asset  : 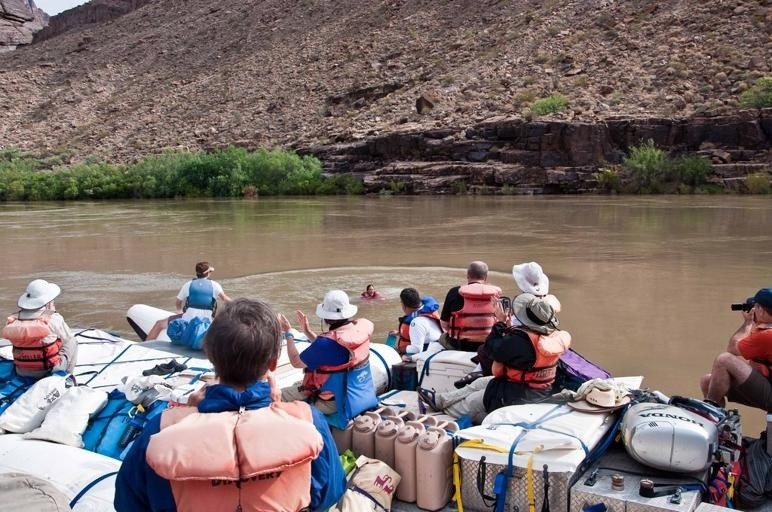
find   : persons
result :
[439,259,504,352]
[144,261,234,341]
[112,297,349,511]
[453,260,550,389]
[359,283,384,301]
[273,288,374,417]
[416,291,559,424]
[387,287,446,363]
[698,287,771,416]
[6,278,80,378]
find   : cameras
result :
[731,297,755,313]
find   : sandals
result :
[417,386,437,411]
[454,371,483,388]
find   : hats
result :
[567,387,631,414]
[16,278,62,310]
[315,289,358,321]
[745,287,772,307]
[511,260,560,335]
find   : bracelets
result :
[284,330,295,339]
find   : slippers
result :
[142,359,188,376]
